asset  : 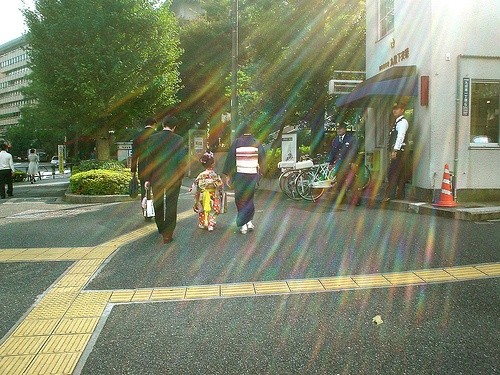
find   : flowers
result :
[204,150,214,159]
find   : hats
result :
[392,102,406,108]
[335,122,347,129]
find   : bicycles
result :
[277,151,372,202]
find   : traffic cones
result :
[431,163,461,208]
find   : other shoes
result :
[240,229,247,234]
[163,233,172,243]
[208,226,213,231]
[199,223,205,228]
[248,224,255,229]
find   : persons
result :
[381,100,409,203]
[130,117,159,221]
[27,148,39,183]
[0,144,16,199]
[327,119,361,207]
[223,122,266,235]
[144,115,185,243]
[190,149,231,232]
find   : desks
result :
[14,165,54,183]
[50,163,74,179]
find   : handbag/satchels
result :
[142,188,155,218]
[129,173,138,198]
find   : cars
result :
[51,156,66,166]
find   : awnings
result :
[334,64,419,109]
